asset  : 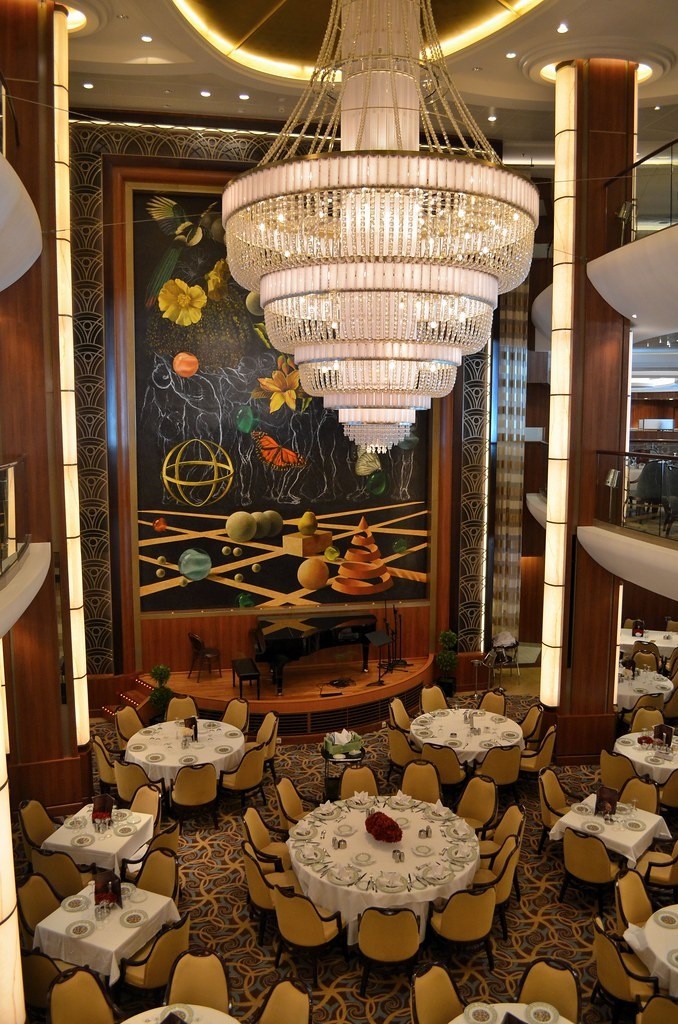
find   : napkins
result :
[380,871,402,886]
[455,818,469,837]
[316,799,337,816]
[425,860,444,880]
[295,819,312,836]
[455,844,471,858]
[334,866,353,881]
[351,790,369,804]
[393,789,411,804]
[302,846,319,861]
[432,798,448,817]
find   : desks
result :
[492,637,520,677]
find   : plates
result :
[615,805,632,815]
[625,819,646,831]
[179,755,197,765]
[526,1002,559,1024]
[112,810,129,821]
[644,756,665,765]
[667,949,678,969]
[215,745,233,754]
[225,730,241,738]
[159,1003,193,1024]
[204,722,218,729]
[463,1002,497,1024]
[64,816,87,829]
[571,802,593,815]
[129,743,146,752]
[289,796,478,892]
[63,895,89,912]
[653,911,678,929]
[145,753,164,762]
[581,821,604,834]
[121,882,137,898]
[66,919,95,938]
[72,833,95,847]
[616,738,635,746]
[139,728,156,736]
[634,688,649,695]
[656,684,671,691]
[416,709,520,749]
[114,824,137,836]
[120,910,148,927]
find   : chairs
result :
[662,465,678,537]
[14,617,678,1024]
[628,460,664,522]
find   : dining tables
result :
[615,667,675,711]
[287,794,482,962]
[623,904,678,998]
[611,731,678,784]
[409,707,526,769]
[548,792,672,872]
[125,717,246,807]
[31,879,181,1016]
[619,628,678,663]
[448,1003,573,1024]
[121,1003,242,1024]
[41,802,154,882]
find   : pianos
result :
[249,614,378,696]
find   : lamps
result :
[220,0,540,458]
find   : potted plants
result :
[436,629,458,699]
[148,663,172,726]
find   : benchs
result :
[231,658,260,699]
